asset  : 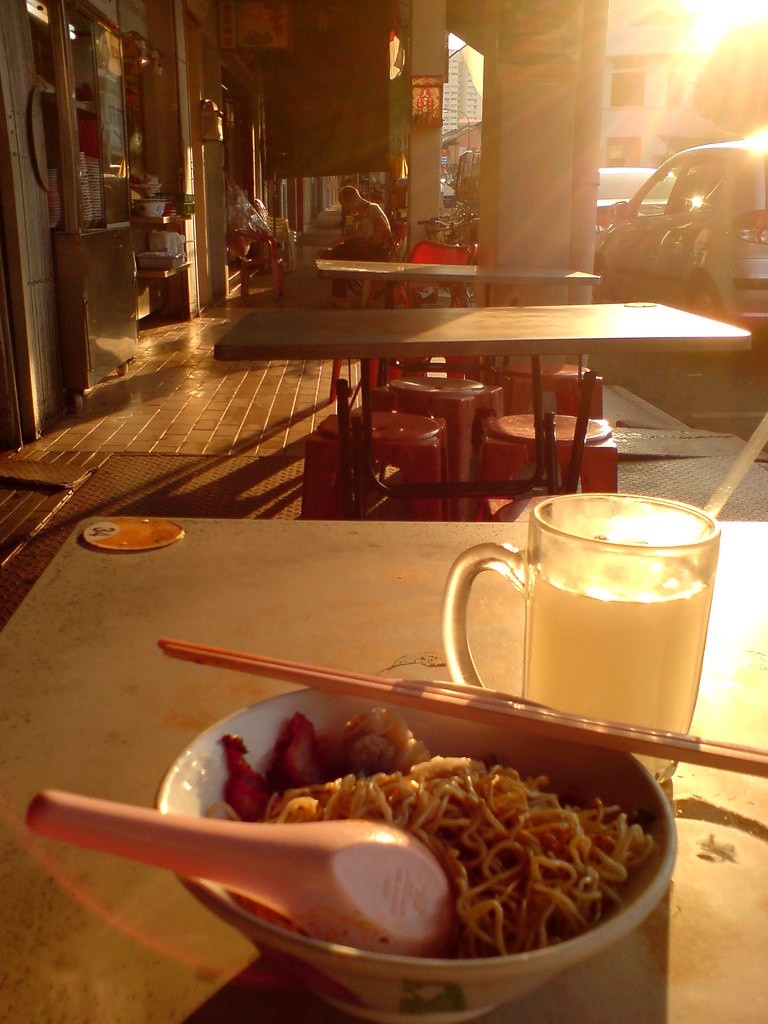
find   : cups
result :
[441,492,721,785]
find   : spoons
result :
[24,786,457,959]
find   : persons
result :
[321,186,394,309]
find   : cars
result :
[595,137,767,330]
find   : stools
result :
[295,356,619,523]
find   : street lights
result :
[441,108,471,150]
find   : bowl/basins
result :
[153,680,677,1024]
[47,151,99,228]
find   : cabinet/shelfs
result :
[132,215,193,279]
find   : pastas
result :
[202,762,653,957]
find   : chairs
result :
[341,223,409,308]
[408,239,477,310]
[227,226,284,298]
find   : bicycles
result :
[415,200,479,308]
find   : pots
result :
[133,198,168,216]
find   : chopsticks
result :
[158,637,768,780]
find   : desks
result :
[0,515,768,1024]
[218,303,754,523]
[313,258,600,306]
